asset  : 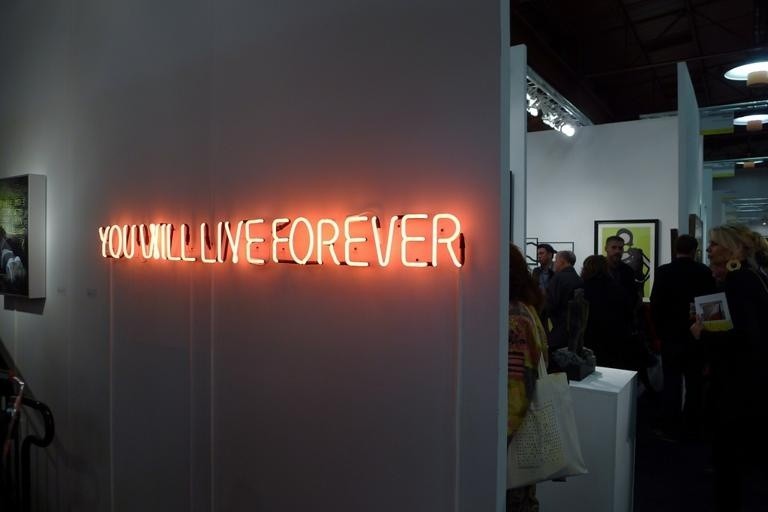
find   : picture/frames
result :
[591,216,661,308]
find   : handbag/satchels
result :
[505,297,590,492]
